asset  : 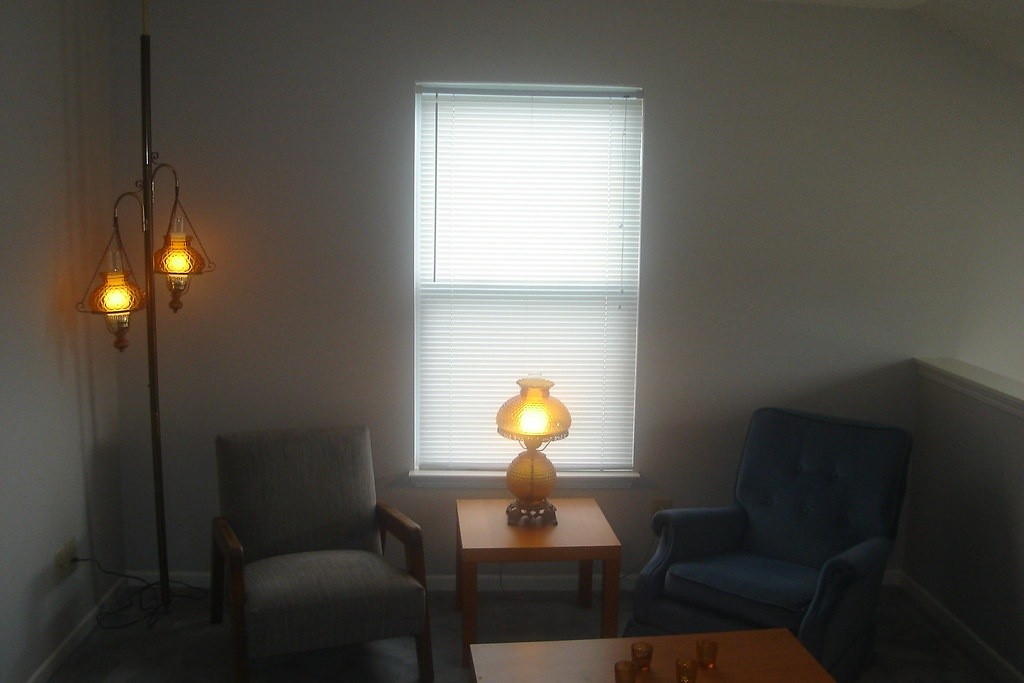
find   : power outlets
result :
[53,538,81,586]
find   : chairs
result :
[211,426,435,683]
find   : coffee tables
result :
[470,627,834,683]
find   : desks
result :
[456,498,622,667]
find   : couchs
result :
[622,407,911,673]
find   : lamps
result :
[77,34,216,606]
[497,378,571,526]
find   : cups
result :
[615,660,637,683]
[696,640,717,668]
[631,642,653,670]
[676,658,698,683]
